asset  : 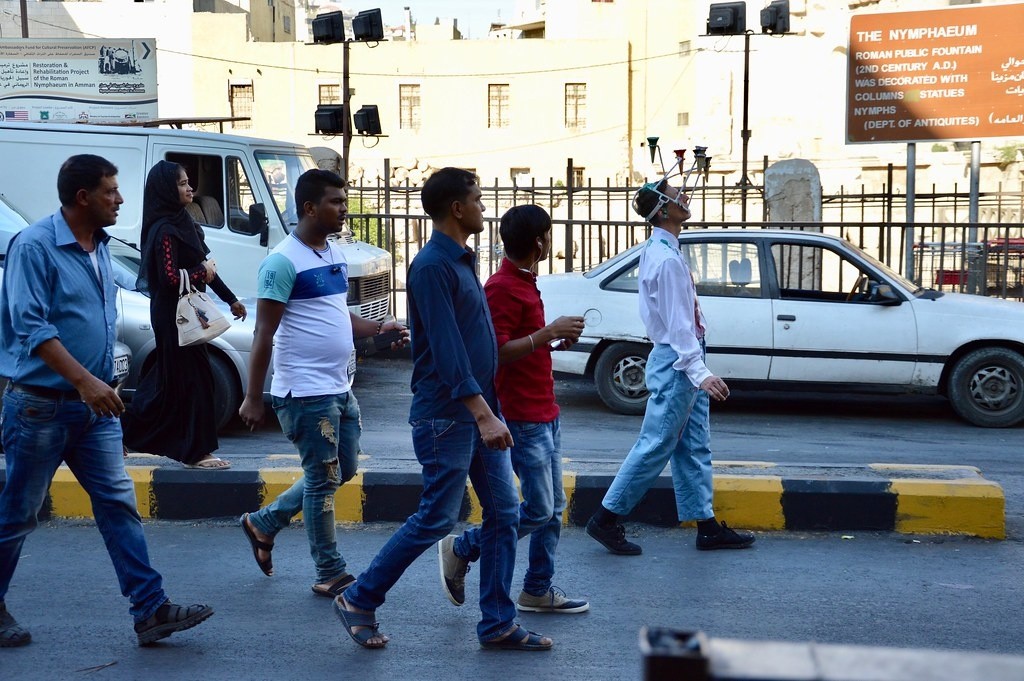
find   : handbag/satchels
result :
[176,269,232,347]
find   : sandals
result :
[134,599,214,645]
[0,616,31,647]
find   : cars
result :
[0,193,290,433]
[537,228,1023,428]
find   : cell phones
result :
[373,329,404,351]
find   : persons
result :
[121,160,247,469]
[436,204,592,615]
[237,170,409,597]
[0,153,216,649]
[333,169,553,651]
[586,180,757,558]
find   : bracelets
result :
[376,321,385,335]
[528,335,535,353]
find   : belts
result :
[14,378,119,401]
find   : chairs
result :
[193,196,223,226]
[729,258,753,297]
[186,202,208,225]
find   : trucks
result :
[0,119,393,355]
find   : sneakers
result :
[584,516,642,555]
[696,520,756,551]
[437,534,470,606]
[517,585,589,613]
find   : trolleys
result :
[909,240,985,296]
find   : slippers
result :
[311,574,357,597]
[479,624,553,651]
[240,512,274,576]
[183,458,231,469]
[331,595,386,648]
